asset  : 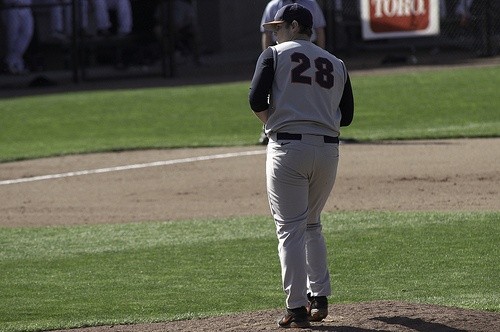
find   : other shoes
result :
[279,306,308,328]
[256,136,269,145]
[308,292,328,322]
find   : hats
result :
[263,3,314,35]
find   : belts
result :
[276,131,339,144]
